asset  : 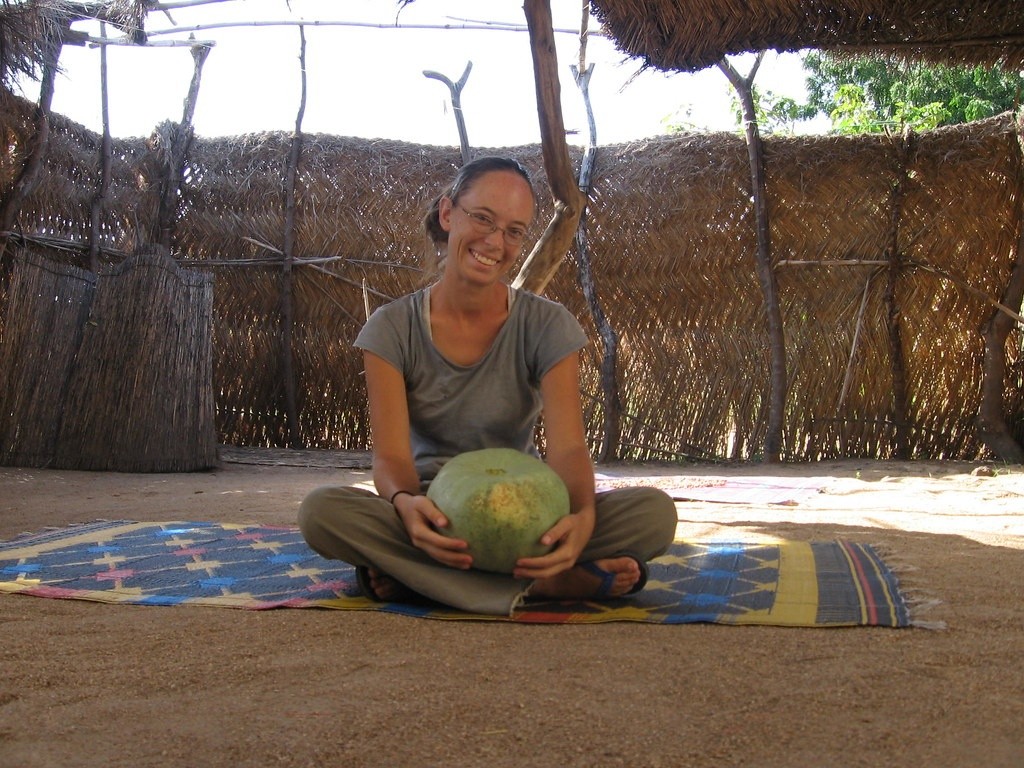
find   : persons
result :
[296,157,677,619]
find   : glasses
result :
[452,199,530,247]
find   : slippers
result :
[355,565,416,603]
[576,552,649,602]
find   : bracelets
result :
[391,491,415,504]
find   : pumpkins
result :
[425,446,570,573]
